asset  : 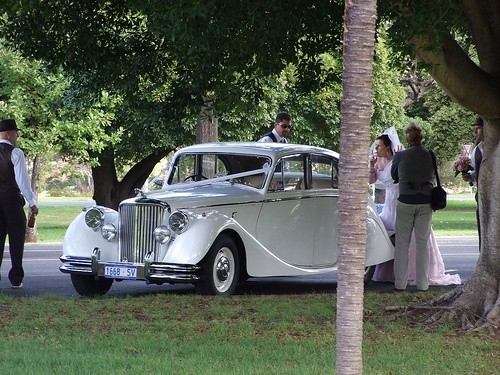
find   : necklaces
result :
[383,157,388,164]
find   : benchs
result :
[239,171,338,195]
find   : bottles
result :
[28,210,35,227]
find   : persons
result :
[257,113,292,184]
[0,119,38,288]
[367,117,483,290]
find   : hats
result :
[0,119,21,131]
[471,117,484,126]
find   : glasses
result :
[277,122,292,129]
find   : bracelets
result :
[370,170,376,174]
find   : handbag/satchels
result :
[431,186,446,211]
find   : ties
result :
[280,141,282,143]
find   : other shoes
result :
[11,278,24,289]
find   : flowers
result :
[453,156,475,186]
